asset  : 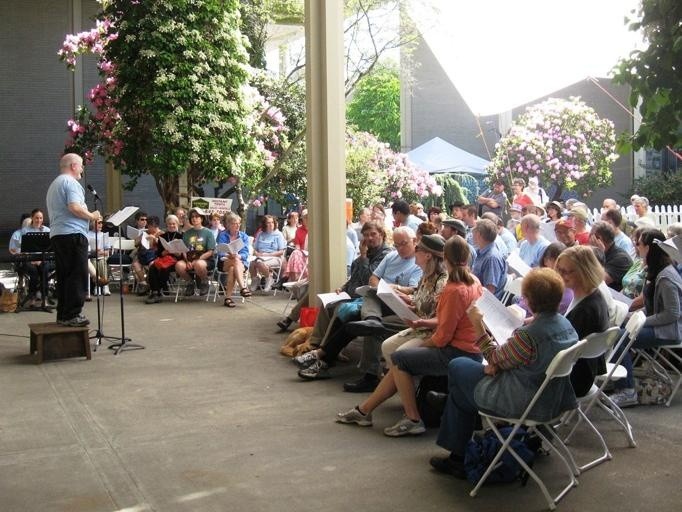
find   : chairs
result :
[341,270,682,474]
[469,338,589,510]
[5,215,310,319]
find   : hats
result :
[442,218,466,235]
[546,201,563,213]
[187,206,207,220]
[421,234,447,258]
[508,203,522,213]
[554,220,576,231]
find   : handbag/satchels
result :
[298,305,321,329]
[626,362,673,405]
[467,425,533,486]
[412,374,447,428]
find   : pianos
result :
[1,251,99,263]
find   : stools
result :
[24,321,92,366]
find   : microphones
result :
[87,184,101,200]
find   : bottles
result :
[122,281,129,293]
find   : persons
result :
[6,206,271,310]
[505,178,535,221]
[476,179,510,224]
[522,175,550,211]
[44,151,104,329]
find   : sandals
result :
[223,297,236,307]
[239,288,252,298]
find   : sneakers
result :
[184,281,210,298]
[293,349,331,380]
[383,415,427,437]
[56,313,91,327]
[344,377,383,393]
[250,276,274,293]
[429,450,465,480]
[278,316,293,329]
[135,283,165,304]
[335,404,375,428]
[94,278,112,297]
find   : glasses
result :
[140,218,148,221]
[603,387,638,408]
[394,240,408,247]
[414,245,425,253]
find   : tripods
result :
[25,250,58,312]
[108,227,147,354]
[89,201,131,351]
